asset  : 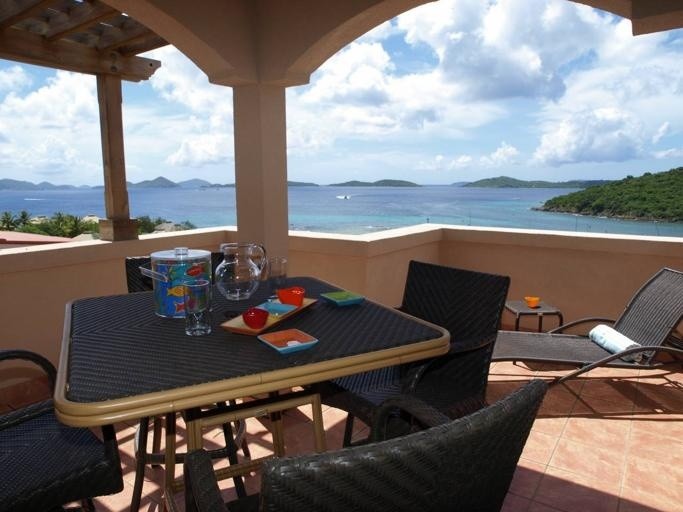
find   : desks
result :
[50,274,453,511]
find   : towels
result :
[588,323,642,362]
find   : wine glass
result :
[182,280,211,337]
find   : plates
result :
[219,292,365,355]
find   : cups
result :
[276,286,306,307]
[523,297,540,308]
[268,255,287,287]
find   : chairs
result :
[1,346,126,511]
[124,253,239,511]
[325,251,513,449]
[182,377,547,512]
[491,267,683,390]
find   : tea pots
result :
[213,241,266,302]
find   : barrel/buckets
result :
[139,248,214,319]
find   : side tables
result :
[505,298,563,365]
